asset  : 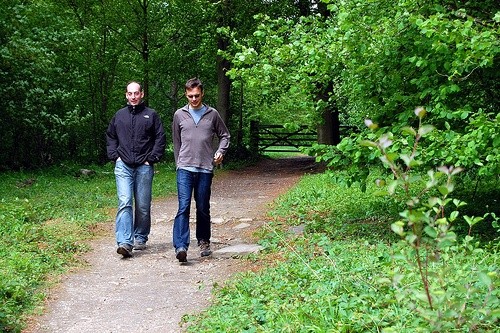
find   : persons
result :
[106,81,167,259]
[172,75,232,262]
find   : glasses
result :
[186,92,203,98]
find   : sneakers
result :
[199,240,211,256]
[117,245,132,257]
[176,248,187,262]
[133,238,146,250]
